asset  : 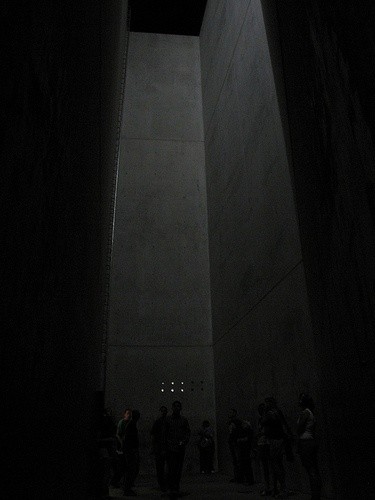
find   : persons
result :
[164,400,191,500]
[118,410,142,497]
[236,419,254,486]
[195,420,216,475]
[106,415,121,451]
[149,406,172,496]
[255,403,272,495]
[110,408,131,488]
[224,407,243,483]
[256,398,296,494]
[286,393,321,500]
